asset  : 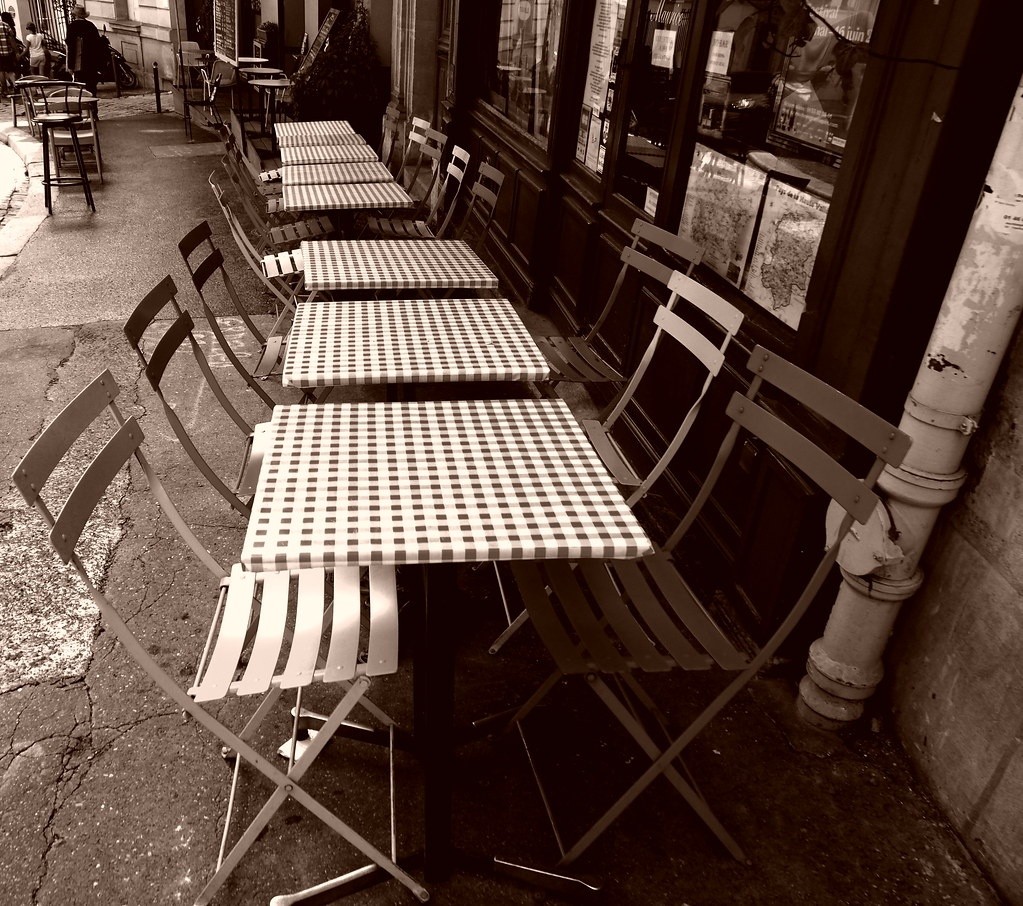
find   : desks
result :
[36,96,99,167]
[236,118,653,906]
[236,56,269,67]
[248,76,295,117]
[251,39,266,69]
[237,68,284,110]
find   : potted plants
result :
[256,21,278,42]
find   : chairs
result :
[498,342,915,873]
[122,274,412,609]
[12,369,431,906]
[200,60,238,115]
[17,82,96,215]
[123,92,708,424]
[176,41,209,100]
[468,269,746,656]
[7,73,105,185]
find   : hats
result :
[71,5,90,18]
[26,21,35,29]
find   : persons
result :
[66,4,103,118]
[0,12,47,98]
